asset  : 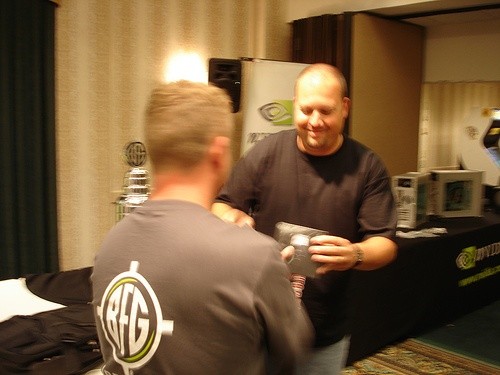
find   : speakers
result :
[208,58,242,113]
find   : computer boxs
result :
[392,172,429,229]
[428,169,483,218]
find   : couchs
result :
[0,266,103,374]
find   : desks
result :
[345,210,500,368]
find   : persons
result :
[212,63,399,375]
[92,80,316,374]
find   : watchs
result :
[353,244,364,270]
